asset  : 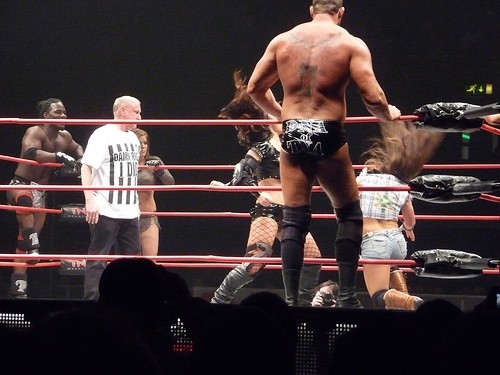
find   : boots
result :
[335,263,364,307]
[210,243,273,304]
[281,269,313,307]
[389,269,409,295]
[383,289,424,310]
[297,264,322,307]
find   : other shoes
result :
[25,248,40,264]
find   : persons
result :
[28,257,298,375]
[330,289,500,375]
[355,118,444,310]
[80,96,141,299]
[312,280,339,308]
[6,99,84,299]
[131,129,174,263]
[209,69,323,307]
[246,0,401,308]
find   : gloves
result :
[56,152,81,177]
